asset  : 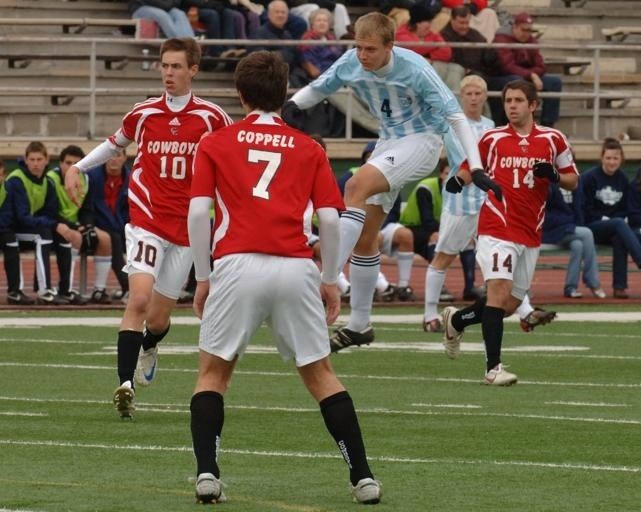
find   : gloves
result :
[473,168,503,201]
[445,176,464,193]
[533,161,561,183]
[282,101,305,127]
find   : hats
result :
[515,12,535,25]
[409,0,441,23]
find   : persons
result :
[337,141,419,303]
[185,1,248,61]
[65,37,234,423]
[285,12,505,357]
[290,0,356,43]
[398,155,484,303]
[442,0,490,11]
[4,141,88,303]
[395,4,453,64]
[438,6,487,71]
[230,0,265,53]
[254,0,297,95]
[541,144,609,300]
[573,138,641,299]
[298,8,347,85]
[442,78,582,387]
[305,133,398,306]
[628,163,641,240]
[88,146,133,304]
[45,144,114,303]
[491,13,563,131]
[185,50,381,504]
[127,0,197,52]
[422,74,559,338]
[0,161,36,304]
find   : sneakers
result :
[569,290,584,298]
[462,286,483,300]
[134,343,158,386]
[8,290,36,305]
[36,290,70,305]
[196,472,228,505]
[350,477,383,503]
[443,306,462,359]
[398,288,417,300]
[592,286,607,298]
[440,285,455,301]
[520,307,555,332]
[484,363,518,386]
[91,287,112,303]
[113,386,135,420]
[423,317,444,331]
[177,285,196,302]
[60,290,89,305]
[376,284,396,302]
[615,288,629,298]
[329,323,375,353]
[340,285,351,303]
